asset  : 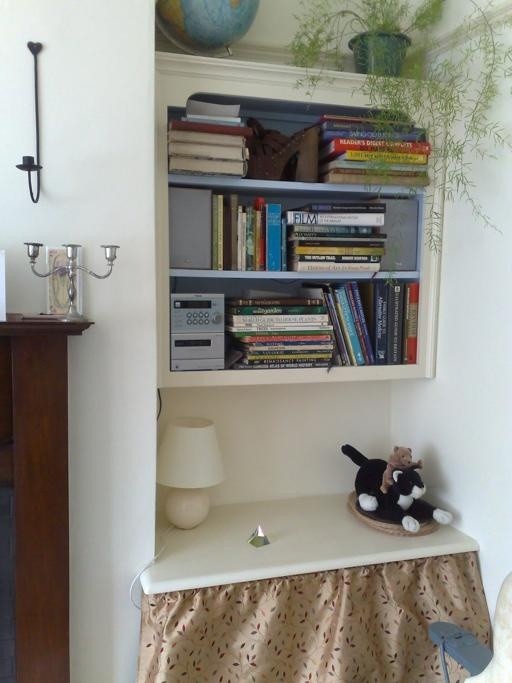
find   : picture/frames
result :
[45,246,82,314]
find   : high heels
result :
[248,126,321,182]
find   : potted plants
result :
[285,0,512,259]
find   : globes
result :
[155,0,260,58]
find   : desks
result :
[140,492,479,594]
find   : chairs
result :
[428,572,512,683]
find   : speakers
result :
[168,186,213,269]
[367,199,419,272]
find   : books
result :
[168,187,287,271]
[282,202,387,272]
[226,299,335,369]
[318,114,432,185]
[166,100,252,178]
[301,280,419,365]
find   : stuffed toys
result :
[379,445,424,494]
[340,443,454,534]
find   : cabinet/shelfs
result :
[0,311,98,683]
[153,49,449,392]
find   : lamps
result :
[155,415,228,531]
[25,243,119,321]
